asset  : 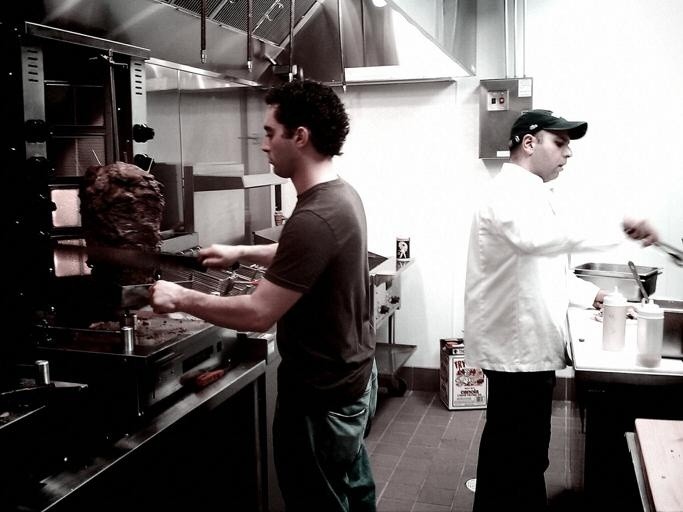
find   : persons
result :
[460,107,665,512]
[146,78,381,510]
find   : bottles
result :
[633,298,665,370]
[601,283,629,353]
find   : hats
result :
[509,110,587,149]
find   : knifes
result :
[141,251,243,276]
